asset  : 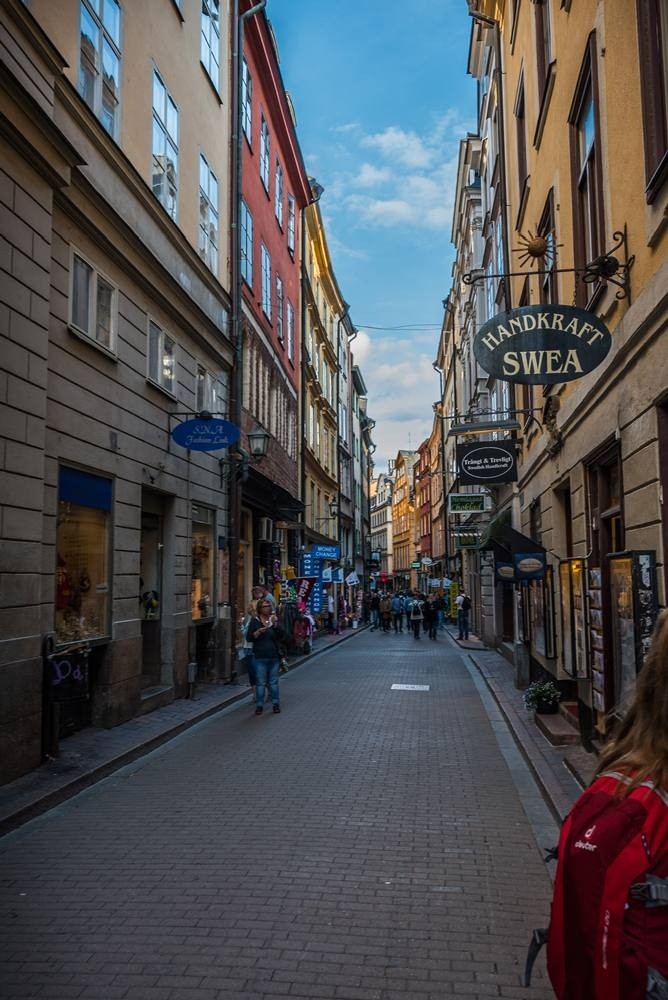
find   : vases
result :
[535,701,560,715]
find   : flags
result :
[320,564,362,587]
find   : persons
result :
[241,599,272,703]
[360,578,471,643]
[543,613,668,1000]
[245,599,286,714]
[251,585,277,614]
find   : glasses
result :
[261,605,272,608]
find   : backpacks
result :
[460,595,471,609]
[413,603,421,616]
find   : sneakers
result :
[256,705,263,713]
[272,705,281,715]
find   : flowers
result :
[522,679,562,712]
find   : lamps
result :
[314,495,339,533]
[219,424,272,488]
[364,530,372,548]
